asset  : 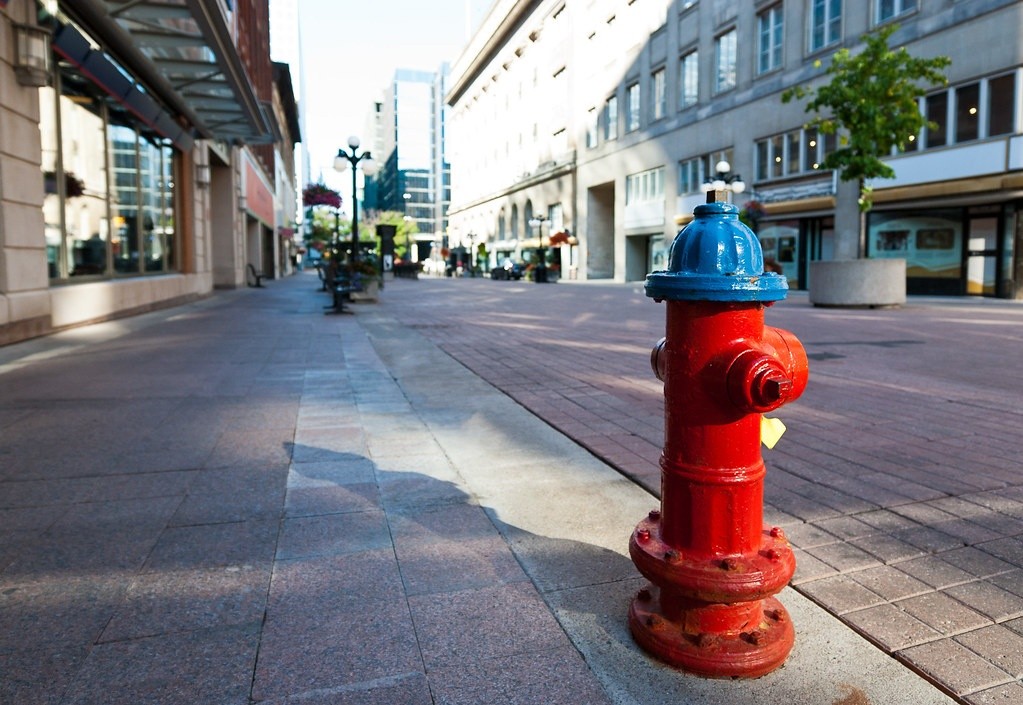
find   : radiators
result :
[526,254,560,281]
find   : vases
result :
[349,281,378,303]
[530,270,559,282]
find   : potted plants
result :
[781,20,953,307]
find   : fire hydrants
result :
[627,190,808,680]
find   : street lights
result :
[333,136,377,267]
[323,206,350,241]
[528,214,552,248]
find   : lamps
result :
[11,21,55,87]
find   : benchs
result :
[315,262,361,315]
[247,263,267,288]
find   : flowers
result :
[349,259,385,293]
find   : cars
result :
[490,257,529,280]
